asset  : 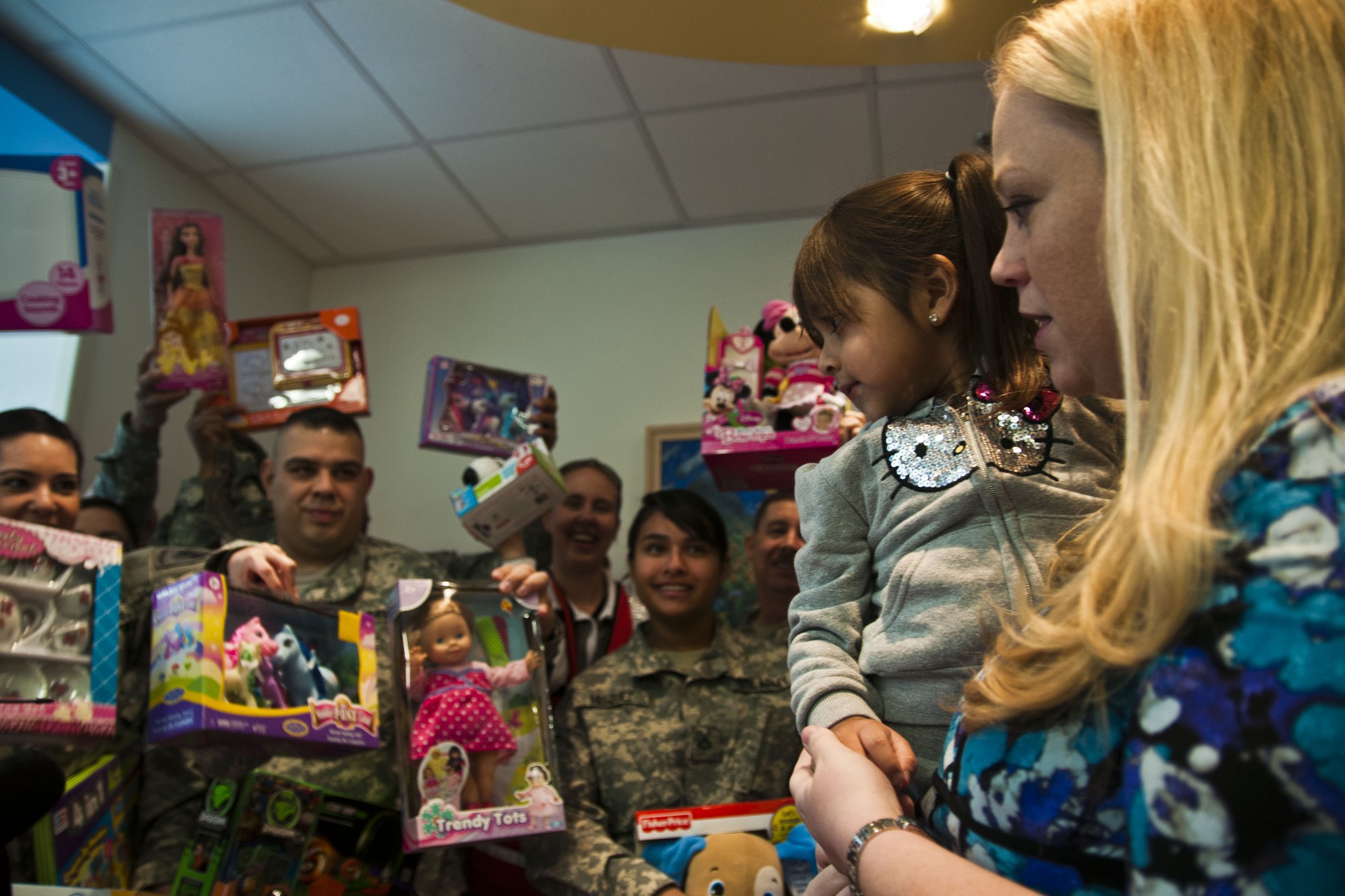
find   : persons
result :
[0,342,567,896]
[535,483,802,896]
[786,145,1125,814]
[749,491,806,652]
[494,458,636,711]
[407,595,541,812]
[791,0,1345,896]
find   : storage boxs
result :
[450,447,564,549]
[384,578,568,850]
[0,152,116,334]
[0,518,123,736]
[419,355,548,456]
[149,572,382,749]
[225,303,373,432]
[172,768,407,896]
[146,209,227,391]
[634,799,817,896]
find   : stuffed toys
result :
[657,823,816,896]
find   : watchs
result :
[847,815,935,896]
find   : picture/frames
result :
[644,422,765,558]
